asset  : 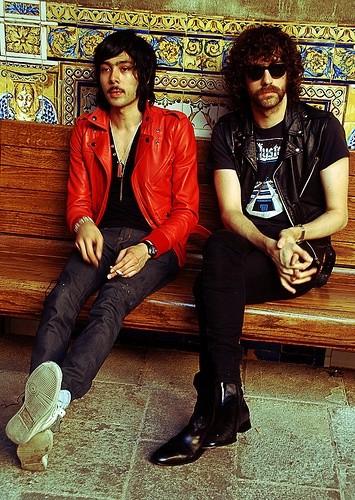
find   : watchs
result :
[295,224,306,244]
[141,239,156,257]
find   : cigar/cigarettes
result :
[109,266,124,276]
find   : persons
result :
[4,33,212,471]
[151,25,349,466]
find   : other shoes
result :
[5,360,71,445]
[17,429,53,471]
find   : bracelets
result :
[73,216,95,233]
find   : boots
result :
[201,381,251,450]
[152,372,213,466]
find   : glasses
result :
[246,63,287,80]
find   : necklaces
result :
[118,138,135,175]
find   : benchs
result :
[0,119,355,351]
